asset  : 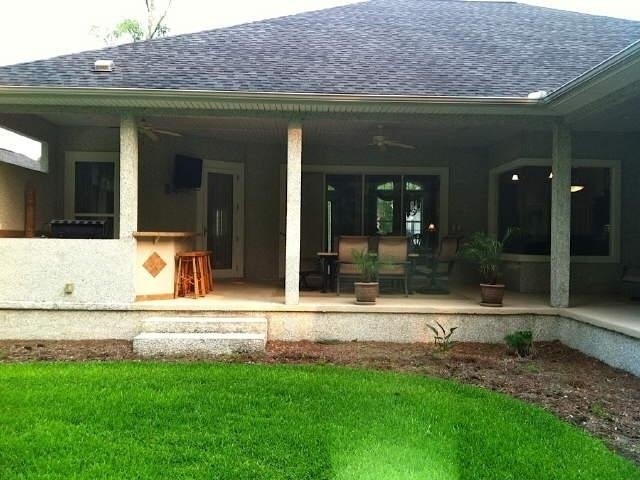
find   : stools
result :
[173,249,213,297]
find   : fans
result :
[355,124,417,150]
[110,119,185,142]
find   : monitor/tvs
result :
[173,154,203,191]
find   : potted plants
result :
[351,248,400,304]
[455,222,523,307]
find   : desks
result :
[316,251,432,293]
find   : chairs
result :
[375,236,411,297]
[426,233,464,284]
[330,235,370,295]
